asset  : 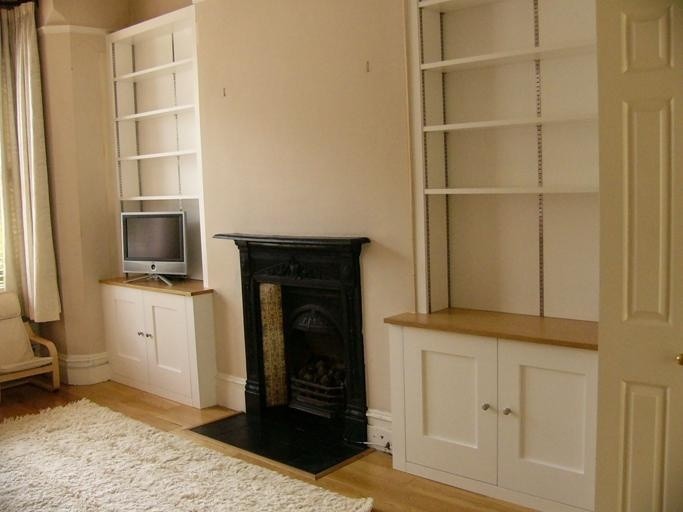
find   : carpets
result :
[0,397,374,512]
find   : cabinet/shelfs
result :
[104,5,199,202]
[101,284,218,410]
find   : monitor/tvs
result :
[119,211,188,288]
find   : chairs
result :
[0,292,60,392]
